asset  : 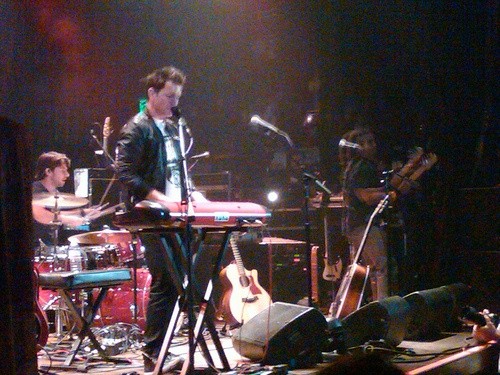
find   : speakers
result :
[330,294,411,349]
[231,301,328,361]
[395,283,457,340]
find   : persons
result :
[31,150,97,247]
[342,128,397,301]
[281,148,318,199]
[118,64,195,373]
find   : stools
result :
[38,269,133,371]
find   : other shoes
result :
[142,348,186,375]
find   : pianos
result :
[111,198,272,375]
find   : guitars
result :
[33,265,49,353]
[328,194,388,319]
[219,234,273,328]
[373,146,437,227]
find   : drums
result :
[85,267,153,334]
[82,234,146,269]
[34,245,87,310]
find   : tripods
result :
[41,202,71,339]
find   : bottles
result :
[68,239,82,273]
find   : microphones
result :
[337,138,366,150]
[250,113,281,133]
[192,151,210,159]
[170,105,189,131]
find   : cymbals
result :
[67,230,132,244]
[31,192,90,207]
